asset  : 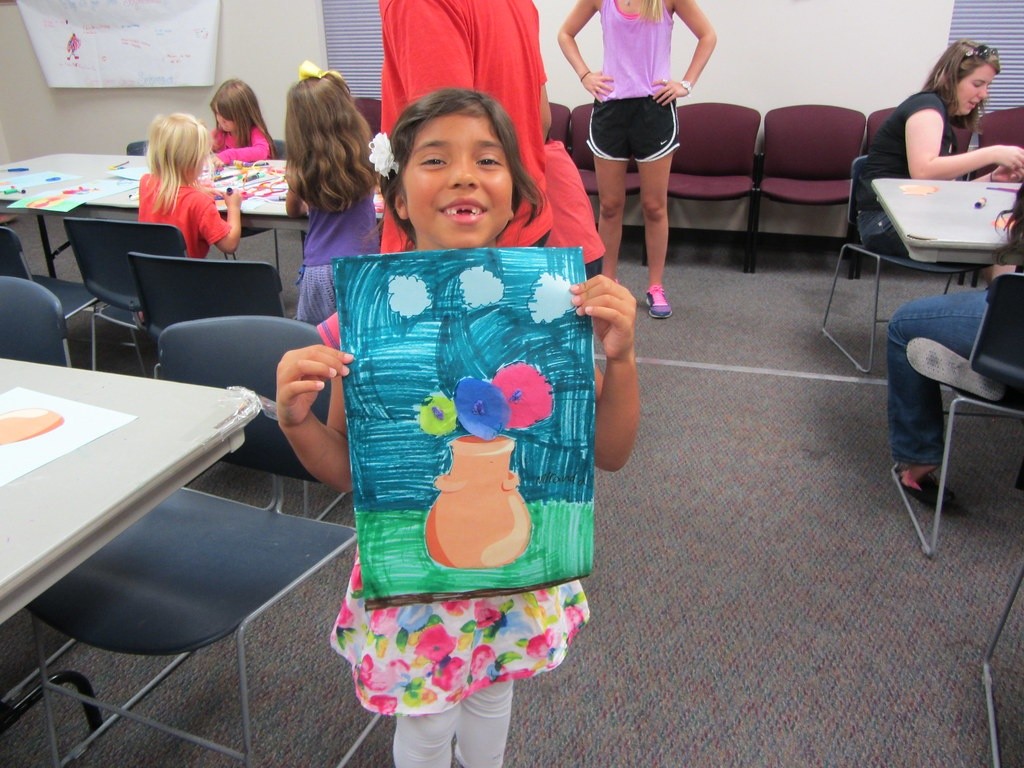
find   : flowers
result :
[368,131,400,181]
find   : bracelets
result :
[581,71,590,82]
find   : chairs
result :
[545,102,570,150]
[667,103,762,273]
[889,272,1024,561]
[748,104,867,281]
[568,102,642,195]
[970,104,1024,288]
[0,225,97,368]
[63,216,187,371]
[823,153,976,373]
[356,98,382,137]
[24,316,383,768]
[127,140,149,156]
[854,106,974,285]
[0,276,73,368]
[224,138,288,275]
[979,561,1024,768]
[126,251,287,339]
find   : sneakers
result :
[615,279,619,284]
[645,284,673,318]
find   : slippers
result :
[906,337,1006,403]
[896,468,955,504]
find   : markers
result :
[227,188,232,195]
[64,189,97,193]
[243,172,265,181]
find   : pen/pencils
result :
[213,175,237,182]
[114,161,130,168]
[0,168,29,172]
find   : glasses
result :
[960,44,998,61]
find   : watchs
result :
[680,80,692,94]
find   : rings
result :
[662,79,668,85]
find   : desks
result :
[0,357,264,768]
[0,153,385,277]
[868,174,1024,265]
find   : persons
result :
[377,0,553,254]
[138,113,242,322]
[557,1,717,317]
[857,38,1024,278]
[210,79,276,167]
[544,128,606,279]
[887,184,1024,508]
[286,61,380,327]
[276,87,641,768]
